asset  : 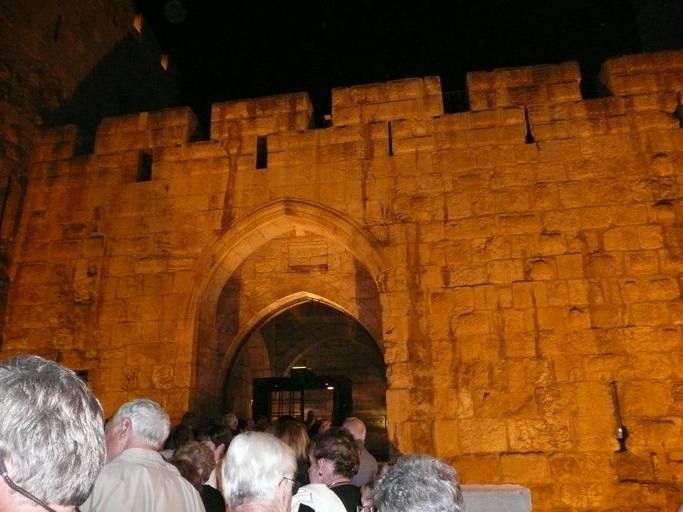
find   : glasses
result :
[0,457,82,512]
[278,477,299,496]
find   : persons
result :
[0,353,467,511]
